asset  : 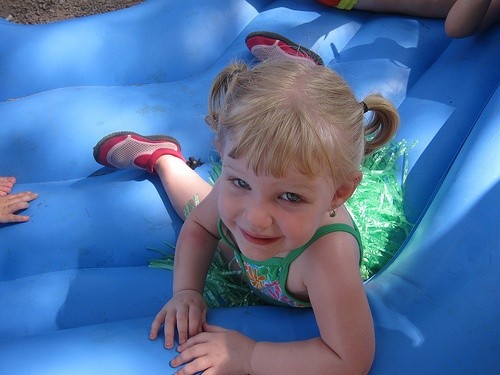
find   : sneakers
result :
[245,30,324,67]
[91,130,187,173]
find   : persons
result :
[318,0,500,39]
[0,173,40,226]
[92,32,414,375]
